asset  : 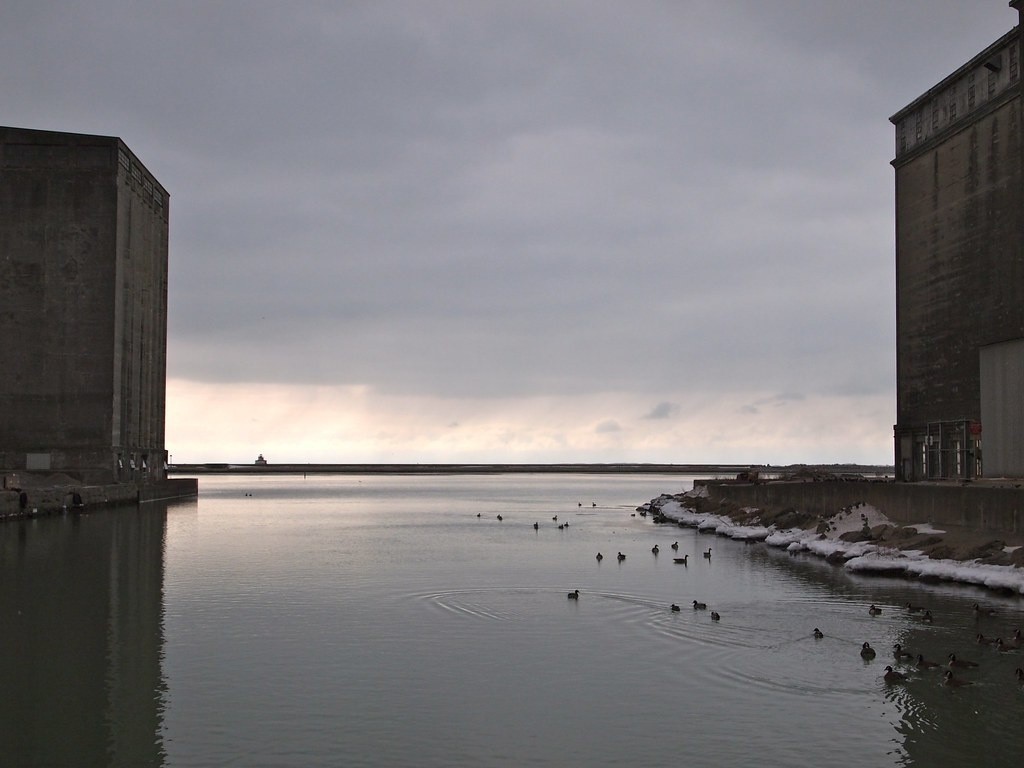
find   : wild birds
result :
[566,590,579,600]
[861,642,876,657]
[578,501,596,506]
[1014,666,1024,685]
[868,604,882,614]
[942,670,977,687]
[921,610,933,622]
[905,602,925,612]
[883,666,910,684]
[651,541,712,563]
[971,603,999,616]
[812,627,824,639]
[596,551,628,564]
[533,514,570,531]
[914,654,940,668]
[891,644,915,660]
[976,627,1024,653]
[477,512,503,521]
[670,598,722,621]
[946,653,979,669]
